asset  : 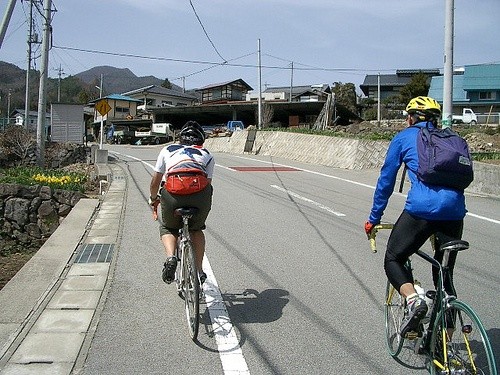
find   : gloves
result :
[365,220,375,236]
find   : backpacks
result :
[399,121,474,193]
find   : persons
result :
[363,96,468,375]
[148,121,215,285]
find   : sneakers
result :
[198,272,207,285]
[162,256,177,284]
[399,298,428,339]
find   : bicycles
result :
[152,196,202,340]
[370,224,497,375]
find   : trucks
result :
[135,123,174,144]
[452,108,478,125]
[202,120,244,139]
[107,125,135,144]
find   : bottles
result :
[424,290,436,317]
[413,279,425,300]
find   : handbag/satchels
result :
[161,168,209,194]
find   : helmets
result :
[406,96,442,120]
[179,120,205,143]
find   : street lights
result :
[95,73,103,98]
